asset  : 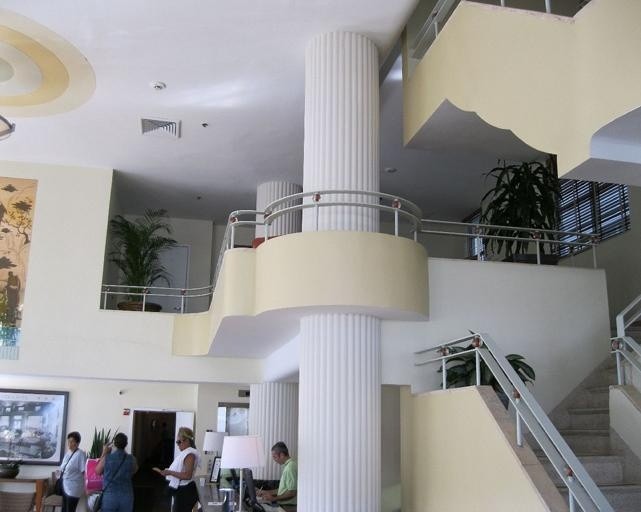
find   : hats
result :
[180,428,194,440]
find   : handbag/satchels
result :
[55,478,63,496]
[93,495,102,512]
[85,458,104,491]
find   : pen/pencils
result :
[258,486,263,496]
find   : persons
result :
[55,431,85,512]
[160,428,199,512]
[255,441,298,512]
[95,432,139,512]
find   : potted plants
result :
[438,329,537,412]
[476,154,564,264]
[106,207,180,313]
[0,459,21,479]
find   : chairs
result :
[35,478,65,512]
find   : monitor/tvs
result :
[241,467,257,506]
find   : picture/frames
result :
[210,456,221,482]
[0,388,70,466]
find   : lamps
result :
[202,431,229,457]
[220,435,260,512]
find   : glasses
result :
[176,438,187,445]
